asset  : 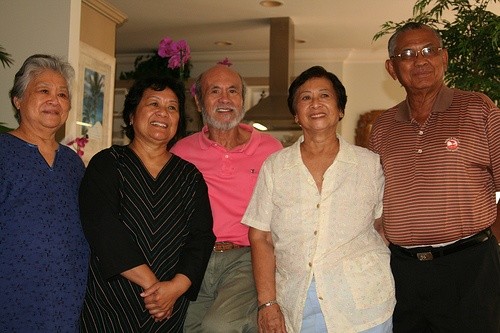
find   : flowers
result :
[117,36,193,81]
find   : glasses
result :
[390,46,443,61]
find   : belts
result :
[388,227,493,262]
[213,239,252,252]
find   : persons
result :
[171,64,283,333]
[369,23,500,333]
[71,69,216,333]
[0,54,92,333]
[239,66,397,333]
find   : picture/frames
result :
[80,64,107,127]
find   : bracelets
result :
[257,299,278,311]
